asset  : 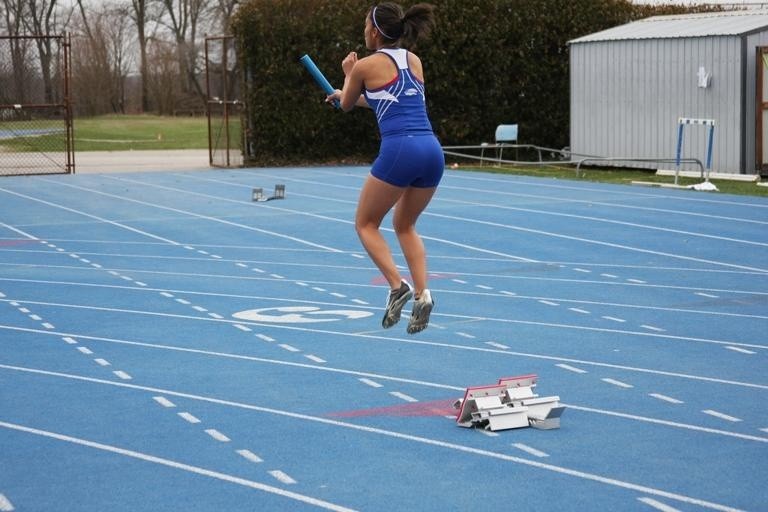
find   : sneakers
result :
[407,289,434,334]
[382,279,413,328]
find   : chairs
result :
[480,123,519,166]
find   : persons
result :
[323,0,445,334]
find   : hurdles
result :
[632,117,716,192]
